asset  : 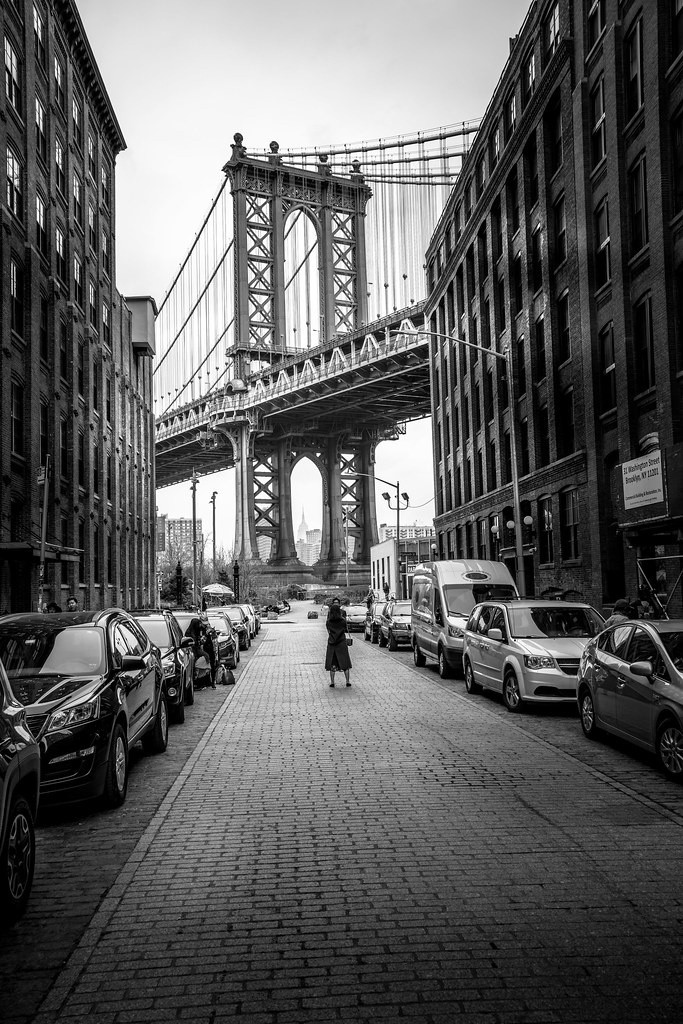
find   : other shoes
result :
[329,684,334,687]
[346,683,351,687]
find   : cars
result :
[206,608,250,651]
[0,659,39,936]
[340,603,369,632]
[225,604,262,639]
[575,618,683,785]
[204,612,240,668]
[0,608,169,809]
[171,611,220,686]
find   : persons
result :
[266,605,279,615]
[382,581,390,601]
[184,598,217,691]
[45,601,62,614]
[325,598,352,688]
[601,598,634,629]
[66,596,78,613]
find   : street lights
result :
[190,467,200,612]
[423,264,427,300]
[154,282,389,419]
[390,328,525,597]
[345,471,409,598]
[402,274,408,307]
[209,491,218,583]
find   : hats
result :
[46,602,62,613]
[615,599,635,610]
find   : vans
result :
[409,560,521,679]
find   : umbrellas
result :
[201,582,235,594]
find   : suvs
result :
[127,610,195,727]
[463,597,609,712]
[378,598,414,651]
[363,600,388,644]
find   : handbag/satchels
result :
[346,638,353,646]
